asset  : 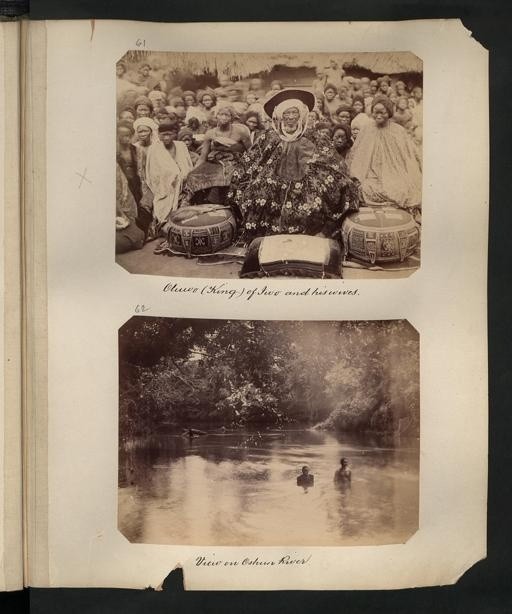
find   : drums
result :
[342,209,418,262]
[166,203,236,256]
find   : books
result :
[2,11,497,597]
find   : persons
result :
[115,56,424,252]
[333,456,352,485]
[295,465,314,488]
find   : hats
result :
[263,89,315,119]
